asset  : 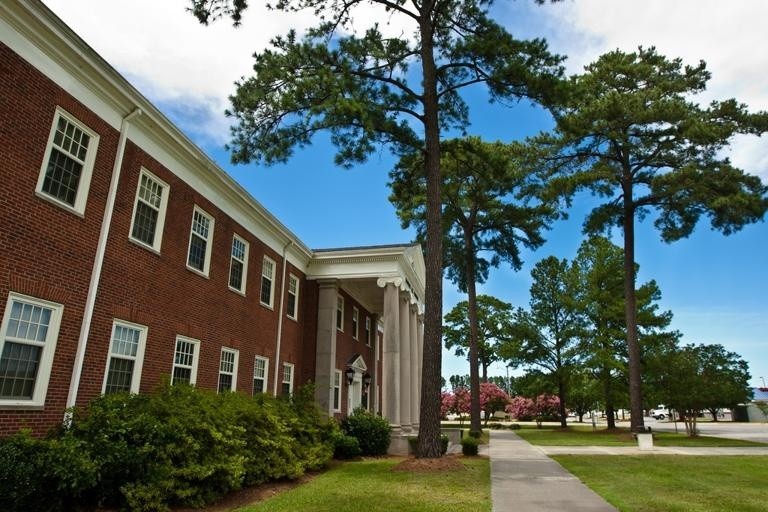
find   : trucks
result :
[648,406,669,421]
